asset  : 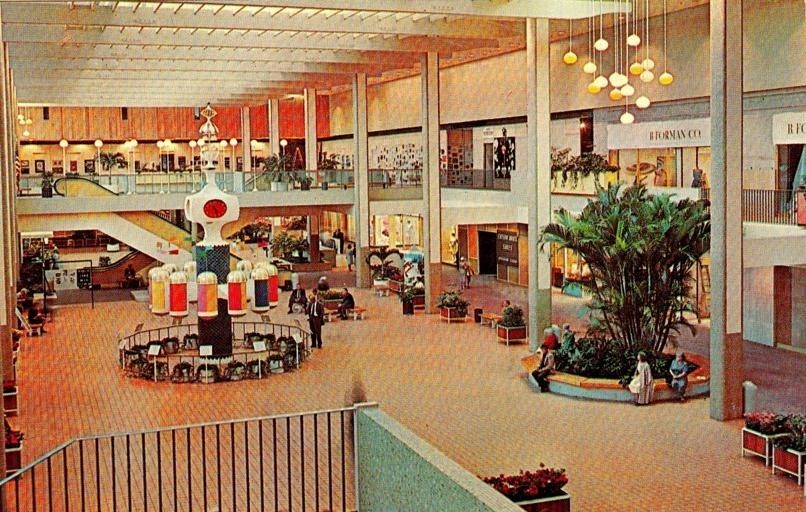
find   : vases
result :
[389,279,403,294]
[772,445,806,484]
[496,325,528,347]
[2,386,19,416]
[4,441,23,480]
[411,295,425,313]
[515,490,571,512]
[741,427,792,468]
[440,307,468,323]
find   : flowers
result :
[406,288,424,300]
[742,411,788,435]
[774,414,806,452]
[3,380,17,392]
[498,306,524,326]
[387,267,404,281]
[476,462,568,502]
[436,289,469,314]
[3,424,24,448]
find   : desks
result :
[33,291,58,322]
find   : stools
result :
[19,322,42,337]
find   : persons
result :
[500,300,513,315]
[561,323,575,351]
[124,263,135,289]
[337,228,345,255]
[332,229,337,238]
[670,351,689,403]
[16,288,33,314]
[458,262,466,292]
[287,283,308,315]
[465,265,475,289]
[28,302,48,334]
[303,295,324,349]
[345,243,352,273]
[531,343,556,394]
[337,288,355,320]
[447,233,459,263]
[310,288,325,304]
[542,330,559,351]
[405,219,415,241]
[353,242,356,267]
[629,352,653,406]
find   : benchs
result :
[348,306,366,320]
[117,276,142,290]
[478,314,503,328]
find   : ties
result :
[311,302,315,316]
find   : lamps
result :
[17,107,32,137]
[60,138,68,177]
[280,139,288,180]
[562,0,673,123]
[164,139,171,194]
[250,140,259,192]
[230,138,238,193]
[128,139,138,195]
[123,141,131,195]
[156,141,165,194]
[94,139,102,175]
[189,140,196,193]
[219,139,228,192]
[196,139,205,190]
